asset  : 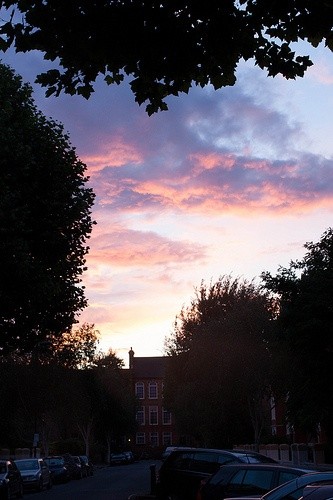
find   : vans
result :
[203,465,317,500]
[158,449,280,498]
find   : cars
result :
[0,460,25,500]
[14,458,53,493]
[224,472,333,500]
[63,456,93,480]
[43,456,70,483]
[110,450,135,465]
